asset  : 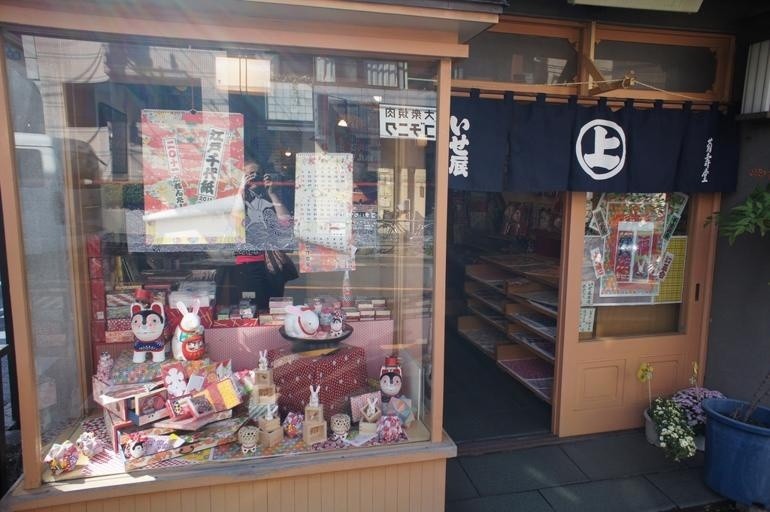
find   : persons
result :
[227,156,295,313]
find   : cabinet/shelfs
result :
[454,251,562,402]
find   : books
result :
[113,252,193,291]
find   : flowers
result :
[638,360,730,463]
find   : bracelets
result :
[273,200,286,207]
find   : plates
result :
[277,319,353,343]
